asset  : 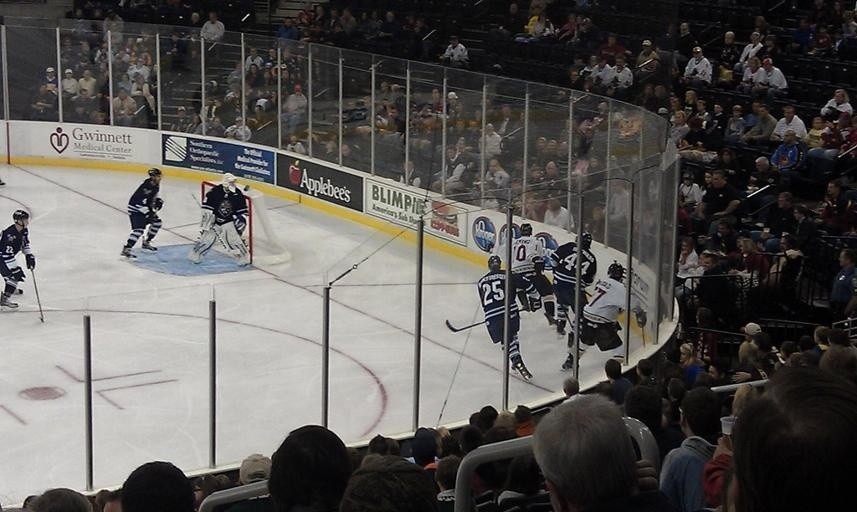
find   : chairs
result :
[197,423,522,512]
[166,0,524,210]
[523,0,856,510]
[1,1,247,124]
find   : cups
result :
[720,416,738,436]
[781,232,789,236]
[762,227,770,233]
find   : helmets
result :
[13,210,28,220]
[222,173,238,193]
[148,167,161,176]
[486,226,624,280]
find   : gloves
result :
[26,254,35,270]
[10,266,26,282]
[145,211,157,226]
[154,197,164,209]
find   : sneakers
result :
[512,304,580,372]
[120,245,138,258]
[0,291,18,307]
[142,236,158,251]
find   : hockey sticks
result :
[446,309,526,332]
[192,194,241,260]
[31,267,44,323]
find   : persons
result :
[189,173,250,268]
[663,1,857,357]
[28,2,662,238]
[550,233,596,335]
[478,255,534,384]
[0,209,36,309]
[18,358,857,512]
[561,263,648,370]
[122,168,165,260]
[511,223,545,311]
